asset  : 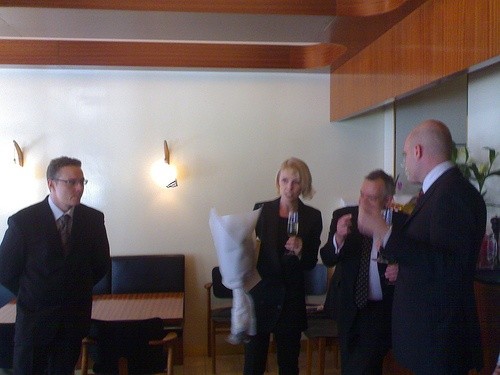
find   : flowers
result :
[452,142,500,196]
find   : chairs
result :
[206,264,339,375]
[81,316,179,375]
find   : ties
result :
[415,189,424,207]
[59,215,71,258]
[355,236,373,311]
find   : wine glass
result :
[286,211,298,257]
[372,207,393,265]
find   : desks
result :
[0,292,185,326]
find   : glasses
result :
[54,178,88,186]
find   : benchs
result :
[0,254,185,365]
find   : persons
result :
[244,158,323,375]
[0,156,110,375]
[320,170,409,375]
[357,121,487,375]
